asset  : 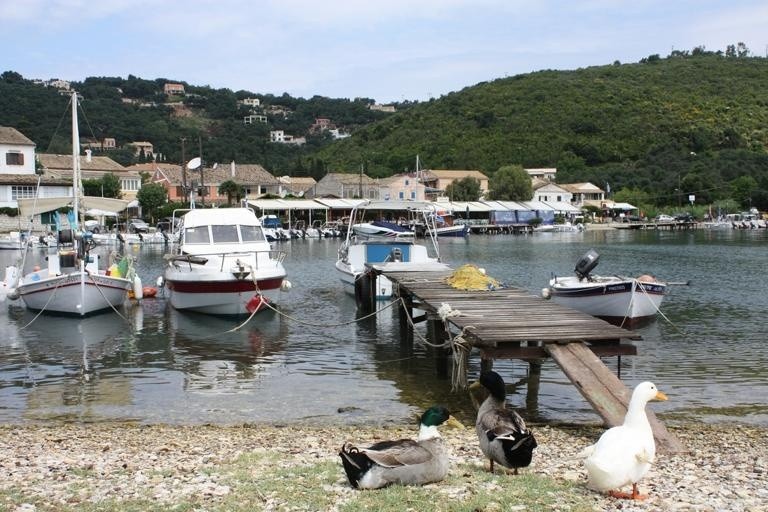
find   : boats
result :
[547,249,668,329]
[335,195,456,302]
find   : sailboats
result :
[2,86,149,319]
[154,205,293,318]
[395,155,471,238]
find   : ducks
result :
[337,406,467,490]
[573,380,670,501]
[465,369,537,476]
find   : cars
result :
[655,214,674,223]
